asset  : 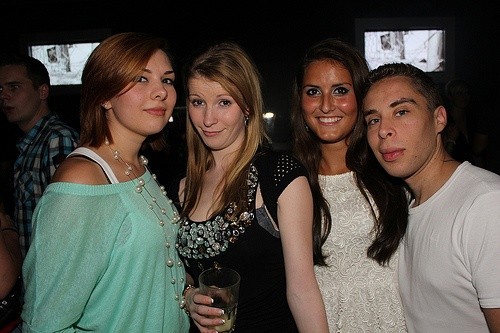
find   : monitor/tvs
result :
[353,17,455,85]
[14,26,111,96]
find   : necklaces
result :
[100,135,186,309]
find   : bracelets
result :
[1,227,19,233]
[182,283,196,318]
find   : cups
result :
[199,269,240,333]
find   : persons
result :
[358,62,500,333]
[157,43,331,333]
[0,211,24,333]
[291,39,412,333]
[1,54,82,260]
[20,30,190,332]
